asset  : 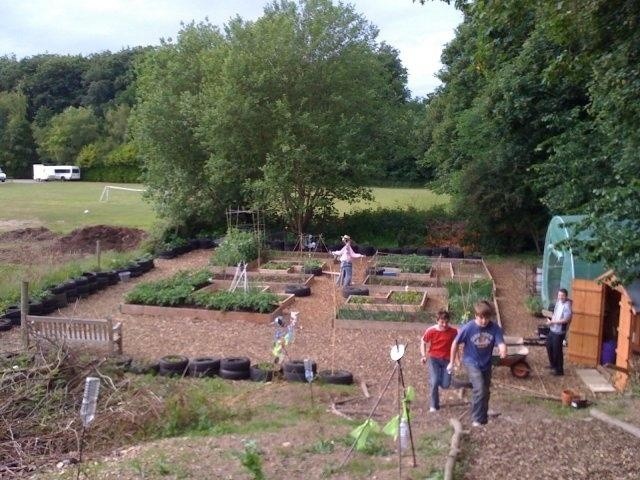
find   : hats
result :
[341,234,352,241]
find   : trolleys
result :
[482,331,546,380]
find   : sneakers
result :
[544,362,565,375]
[428,405,486,430]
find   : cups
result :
[560,389,582,410]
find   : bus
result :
[33,163,81,182]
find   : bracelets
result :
[422,356,427,358]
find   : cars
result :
[0,168,6,181]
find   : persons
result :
[420,310,461,412]
[545,288,574,375]
[447,300,508,425]
[329,235,365,287]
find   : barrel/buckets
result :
[600,337,615,366]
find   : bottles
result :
[78,374,100,429]
[399,418,412,451]
[303,357,315,384]
